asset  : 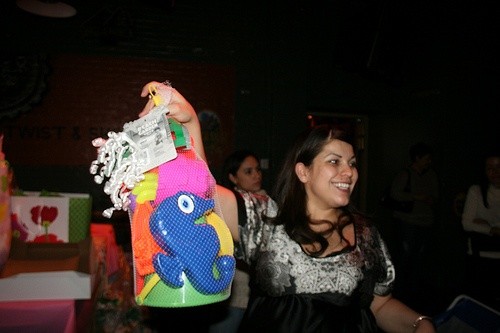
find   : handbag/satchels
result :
[0,190,92,300]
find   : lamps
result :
[16,0,78,18]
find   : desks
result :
[0,234,90,332]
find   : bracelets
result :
[411,316,433,331]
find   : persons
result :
[209,150,272,333]
[139,81,436,333]
[461,153,500,312]
[390,143,438,317]
